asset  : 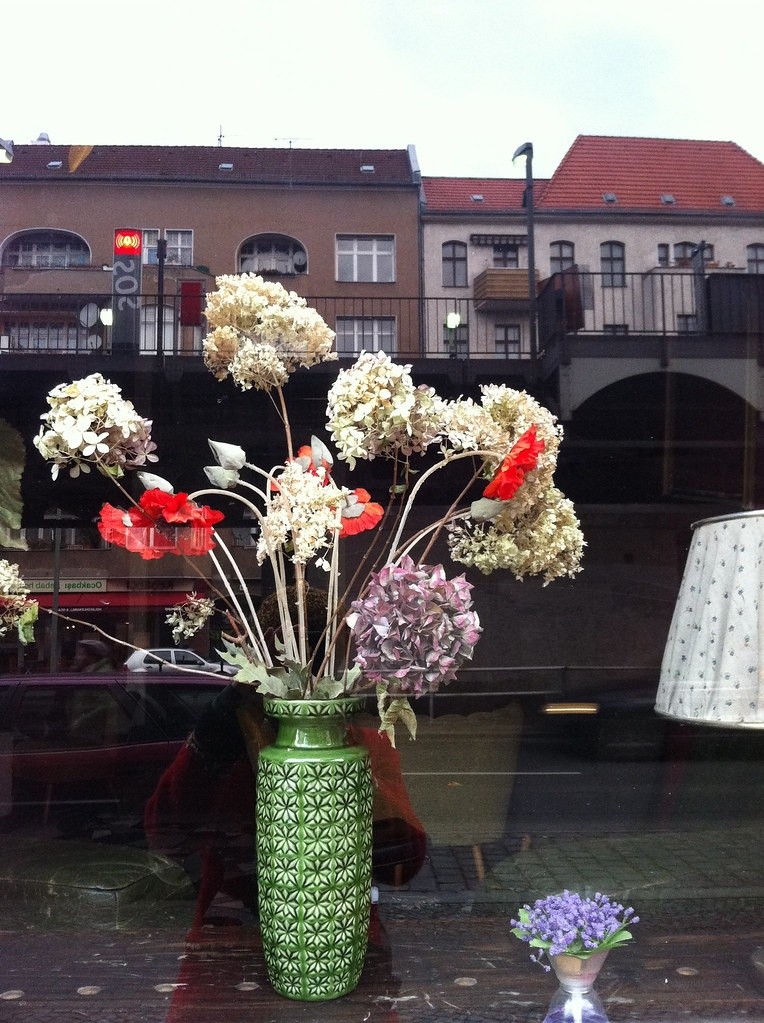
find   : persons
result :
[142,581,430,1022]
[38,636,125,847]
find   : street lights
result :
[508,142,538,401]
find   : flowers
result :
[510,889,640,961]
[0,271,586,746]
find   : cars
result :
[0,675,238,835]
[122,648,242,678]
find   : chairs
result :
[350,702,526,893]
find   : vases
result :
[253,697,376,1002]
[544,951,611,995]
